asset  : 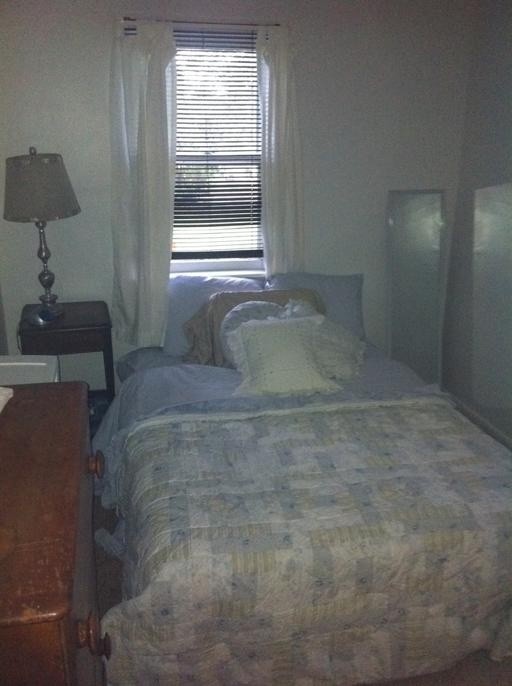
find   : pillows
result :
[163,270,371,400]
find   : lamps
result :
[2,146,83,327]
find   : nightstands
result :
[18,300,116,405]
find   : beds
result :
[108,328,511,684]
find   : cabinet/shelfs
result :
[0,380,107,686]
